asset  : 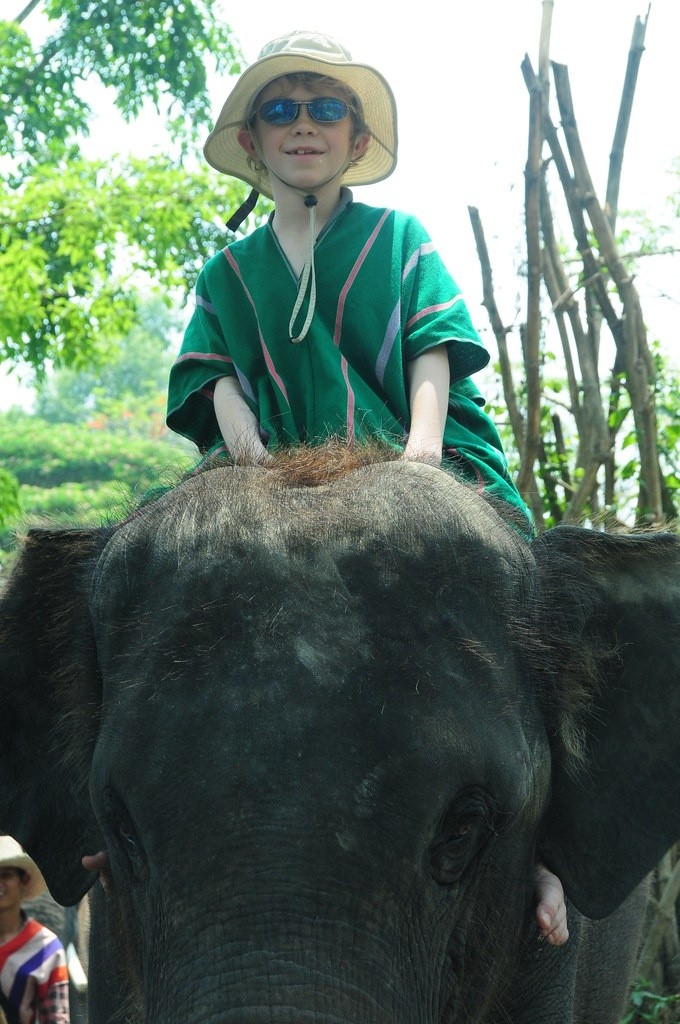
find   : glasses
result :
[250,97,356,125]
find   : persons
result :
[81,32,571,946]
[0,835,70,1024]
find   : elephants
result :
[0,403,680,1024]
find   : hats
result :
[0,836,46,899]
[203,31,398,232]
